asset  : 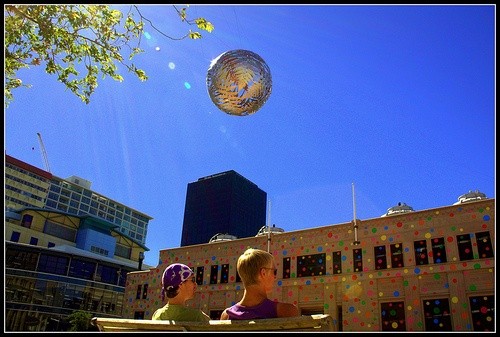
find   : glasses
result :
[260,267,277,275]
[184,277,196,283]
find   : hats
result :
[161,263,193,291]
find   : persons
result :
[220,249,301,320]
[152,263,211,321]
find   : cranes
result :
[36,132,50,174]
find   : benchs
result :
[90,314,337,332]
